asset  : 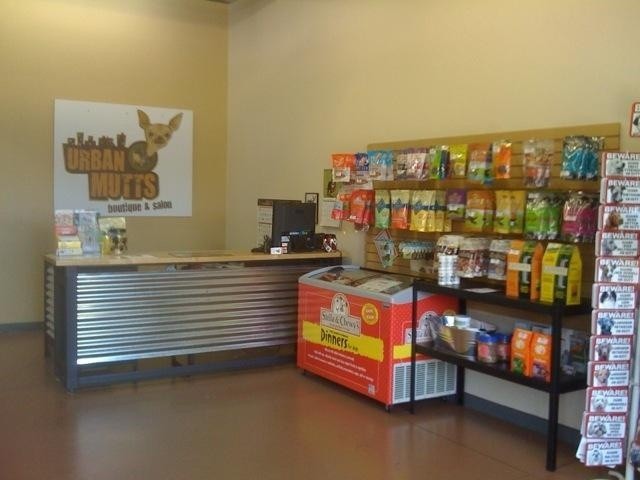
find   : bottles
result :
[108,227,128,258]
[478,332,509,365]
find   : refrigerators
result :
[296,266,463,411]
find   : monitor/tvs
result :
[272,199,316,246]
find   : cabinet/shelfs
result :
[410,278,594,472]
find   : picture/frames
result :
[304,193,319,225]
[322,168,343,202]
